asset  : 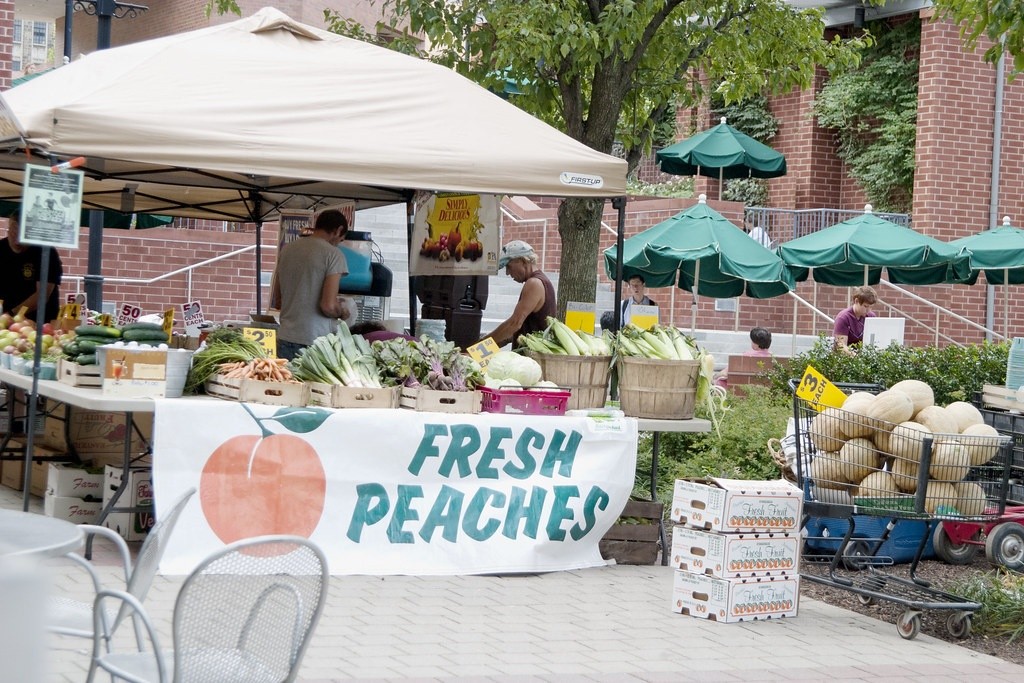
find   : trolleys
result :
[787,377,1014,641]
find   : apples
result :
[0,313,70,355]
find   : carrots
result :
[210,357,301,396]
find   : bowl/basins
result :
[94,345,193,397]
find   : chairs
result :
[85,534,329,683]
[42,486,198,683]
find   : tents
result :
[0,8,629,513]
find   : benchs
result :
[726,353,808,402]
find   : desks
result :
[0,507,87,568]
[0,353,712,568]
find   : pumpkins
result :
[447,223,462,253]
[810,377,1000,515]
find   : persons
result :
[621,275,661,327]
[349,322,418,342]
[713,327,772,396]
[477,241,557,354]
[25,63,35,75]
[600,310,616,335]
[833,286,877,356]
[13,56,70,87]
[745,216,771,249]
[273,209,351,362]
[0,208,64,434]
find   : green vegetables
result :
[22,348,65,363]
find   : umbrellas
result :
[602,195,796,339]
[656,117,788,200]
[0,201,171,229]
[949,216,1024,344]
[775,205,974,287]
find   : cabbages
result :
[465,349,564,392]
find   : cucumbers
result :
[61,322,169,365]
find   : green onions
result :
[289,319,383,400]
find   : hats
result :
[498,239,534,272]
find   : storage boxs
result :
[50,462,106,501]
[304,380,403,409]
[45,491,103,527]
[204,373,311,407]
[0,316,200,541]
[598,493,664,566]
[400,386,483,415]
[670,475,805,624]
[105,463,155,547]
[44,398,154,466]
[0,435,46,493]
[27,440,64,498]
[478,385,572,416]
[56,358,104,390]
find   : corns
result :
[519,317,695,360]
